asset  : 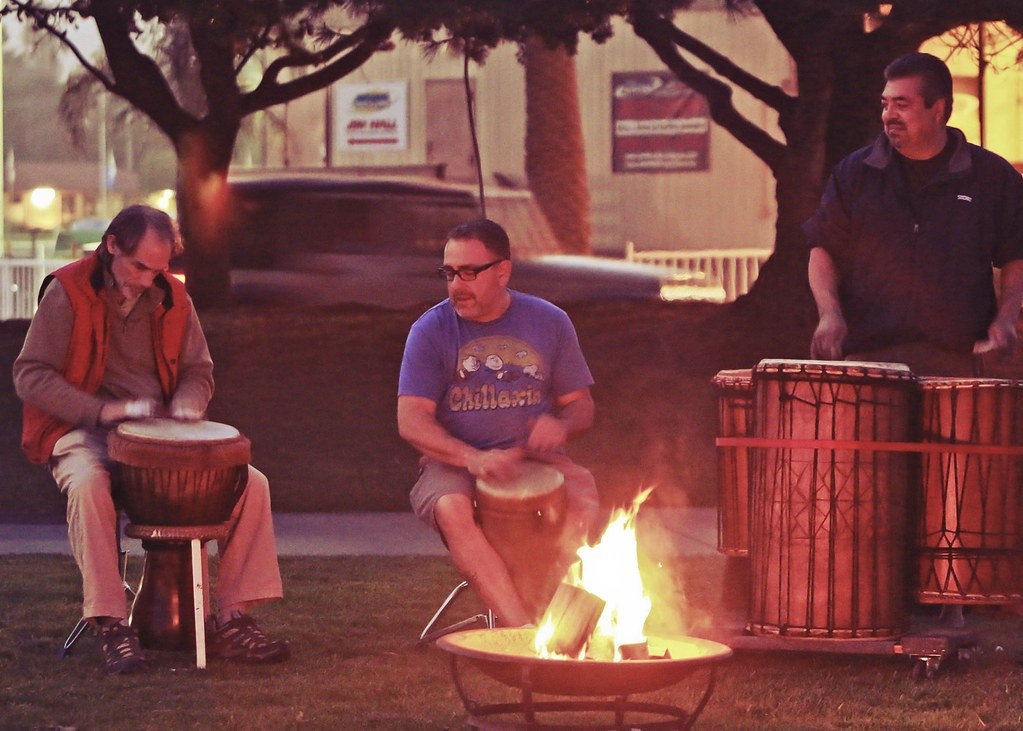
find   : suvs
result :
[214,168,727,312]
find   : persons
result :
[12,205,288,676]
[397,218,600,628]
[801,51,1023,361]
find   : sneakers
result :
[211,611,289,660]
[101,621,144,675]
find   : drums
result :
[104,416,254,666]
[710,355,1021,641]
[475,457,571,630]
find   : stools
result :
[420,533,498,642]
[47,481,146,658]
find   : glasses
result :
[439,260,504,282]
